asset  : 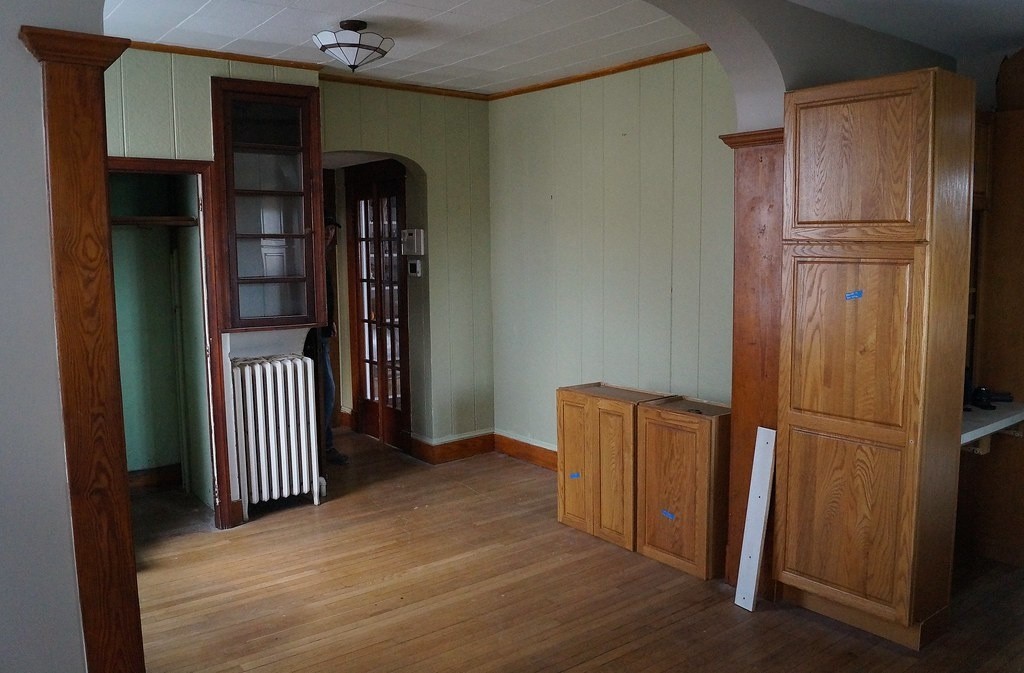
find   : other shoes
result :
[326,448,352,466]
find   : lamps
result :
[311,20,397,73]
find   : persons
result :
[303,212,350,466]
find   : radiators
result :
[235,349,323,521]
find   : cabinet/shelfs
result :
[553,69,1023,655]
